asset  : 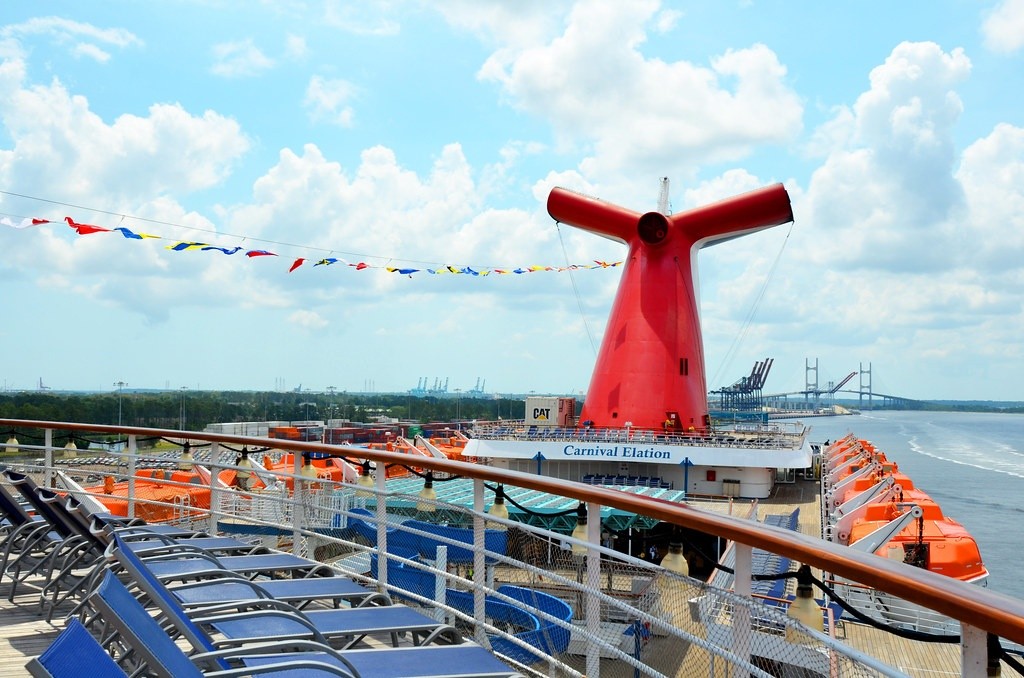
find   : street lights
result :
[407,390,412,421]
[453,389,460,430]
[113,380,129,483]
[179,387,188,444]
[299,402,317,443]
[327,386,337,445]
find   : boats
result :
[825,439,989,595]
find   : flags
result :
[313,258,338,267]
[64,216,113,235]
[1,216,56,230]
[385,267,399,273]
[201,247,244,255]
[246,250,279,258]
[348,263,367,271]
[289,258,305,272]
[427,259,623,277]
[165,240,210,251]
[399,269,420,274]
[114,227,162,240]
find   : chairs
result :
[0,466,409,645]
[88,567,360,676]
[25,615,135,678]
[733,506,846,635]
[112,531,525,676]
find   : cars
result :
[35,448,282,470]
[86,474,101,483]
[12,493,25,503]
[70,476,83,482]
[19,468,25,473]
[56,482,59,487]
[6,466,13,471]
[27,468,33,473]
[34,468,42,473]
